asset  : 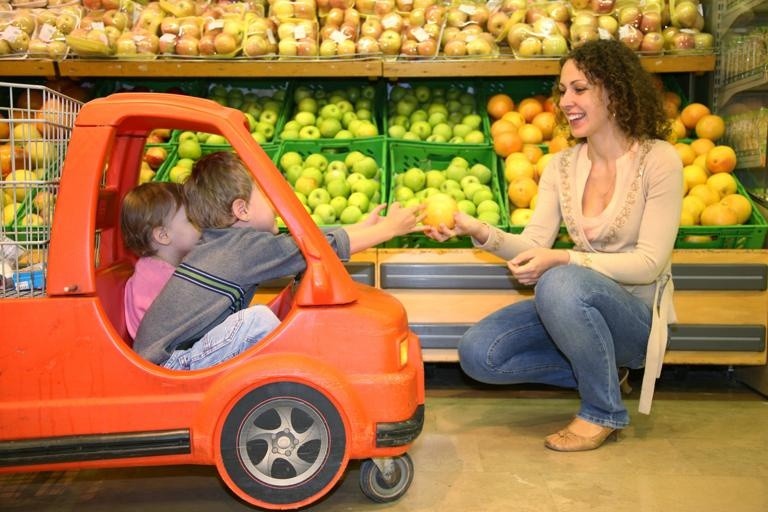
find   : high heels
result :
[618,368,632,394]
[544,427,621,451]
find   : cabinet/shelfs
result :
[0,54,767,366]
[704,2,767,398]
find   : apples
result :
[1,93,70,226]
[273,149,381,229]
[136,86,283,187]
[1,0,715,62]
[280,84,381,141]
[388,81,485,145]
[487,75,752,239]
[395,155,500,234]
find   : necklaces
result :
[587,175,615,199]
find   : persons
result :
[132,149,431,370]
[423,38,683,451]
[119,182,202,341]
